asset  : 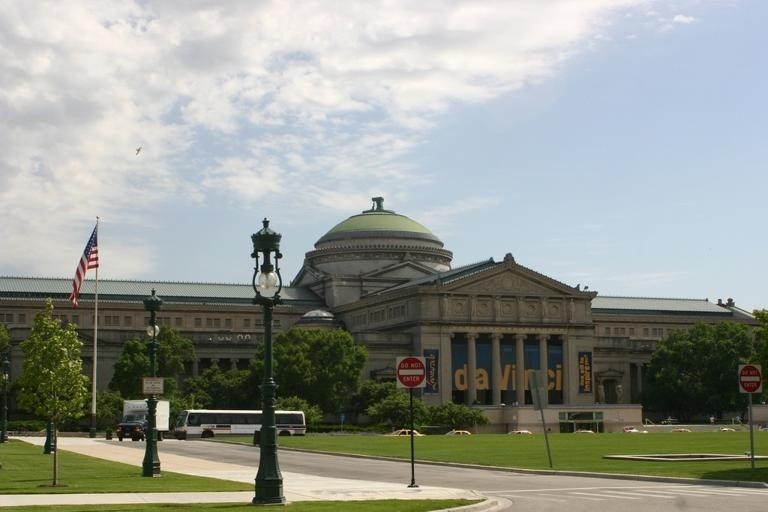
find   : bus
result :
[173,408,306,439]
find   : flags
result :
[69,223,98,311]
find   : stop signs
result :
[395,356,427,388]
[737,363,762,394]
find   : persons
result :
[710,415,715,424]
[736,415,743,424]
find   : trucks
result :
[113,397,173,442]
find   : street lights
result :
[0,357,12,442]
[247,218,288,506]
[139,288,164,476]
